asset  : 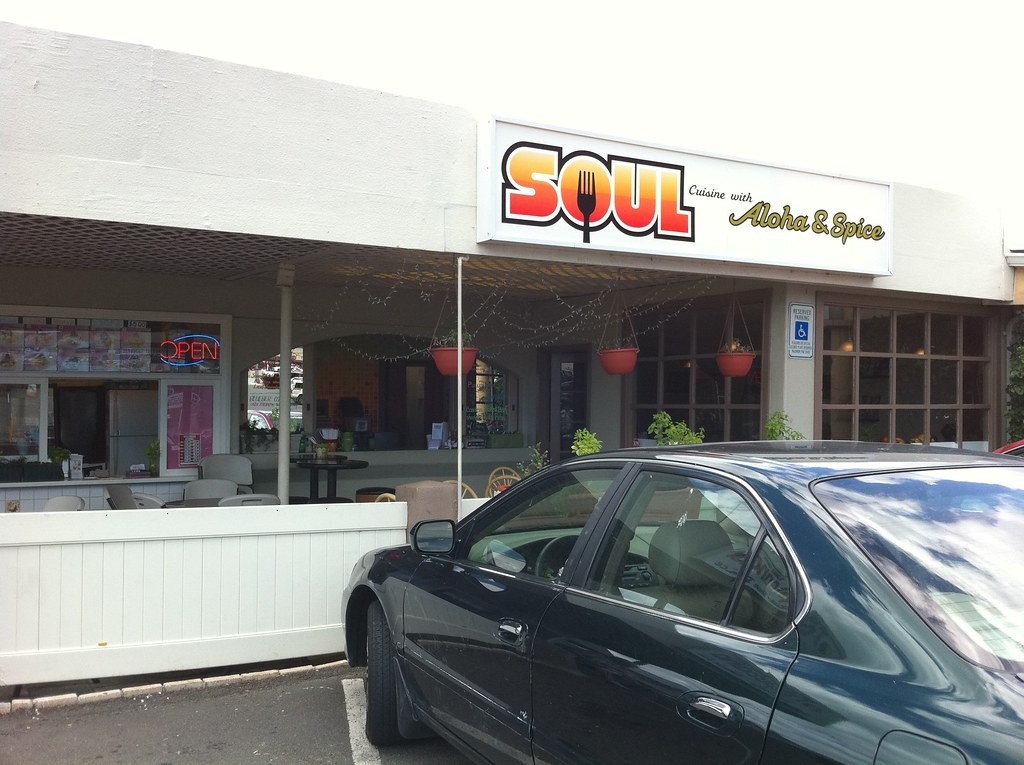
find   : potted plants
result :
[596,335,642,376]
[715,338,758,378]
[430,328,480,381]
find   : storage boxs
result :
[485,434,523,448]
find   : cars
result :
[992,439,1024,459]
[248,409,302,432]
[342,439,1024,765]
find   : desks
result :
[290,454,347,497]
[166,497,227,508]
[297,458,370,497]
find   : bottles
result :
[306,438,312,452]
[299,437,305,452]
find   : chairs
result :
[181,478,240,501]
[630,517,780,631]
[131,491,166,508]
[198,452,258,498]
[102,485,138,510]
[44,495,85,511]
[443,480,478,499]
[488,465,522,497]
[485,476,523,498]
[375,493,396,503]
[216,492,281,506]
